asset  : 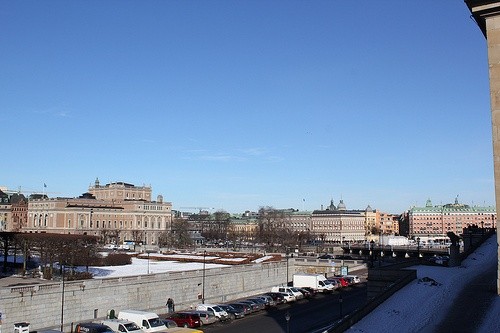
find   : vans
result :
[118,310,168,332]
[271,287,303,300]
[196,305,228,321]
[101,319,143,333]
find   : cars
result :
[163,312,200,330]
[328,280,342,290]
[258,296,274,306]
[219,306,241,320]
[318,255,334,259]
[229,303,244,319]
[339,279,348,288]
[298,288,310,297]
[193,310,215,327]
[301,287,314,295]
[281,292,295,303]
[265,292,284,304]
[240,302,252,316]
[345,277,355,286]
[335,255,352,260]
[74,323,114,333]
[247,299,263,312]
[343,275,360,283]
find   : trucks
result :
[293,274,335,295]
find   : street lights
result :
[60,266,77,332]
[203,251,207,303]
[417,235,420,258]
[286,249,289,286]
[370,239,375,267]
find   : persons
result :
[165,298,176,315]
